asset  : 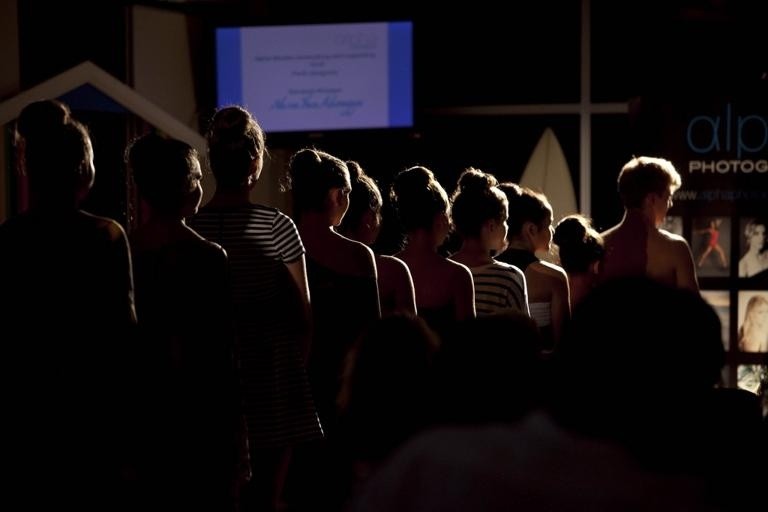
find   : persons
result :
[1,100,768,511]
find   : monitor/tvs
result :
[196,12,421,143]
